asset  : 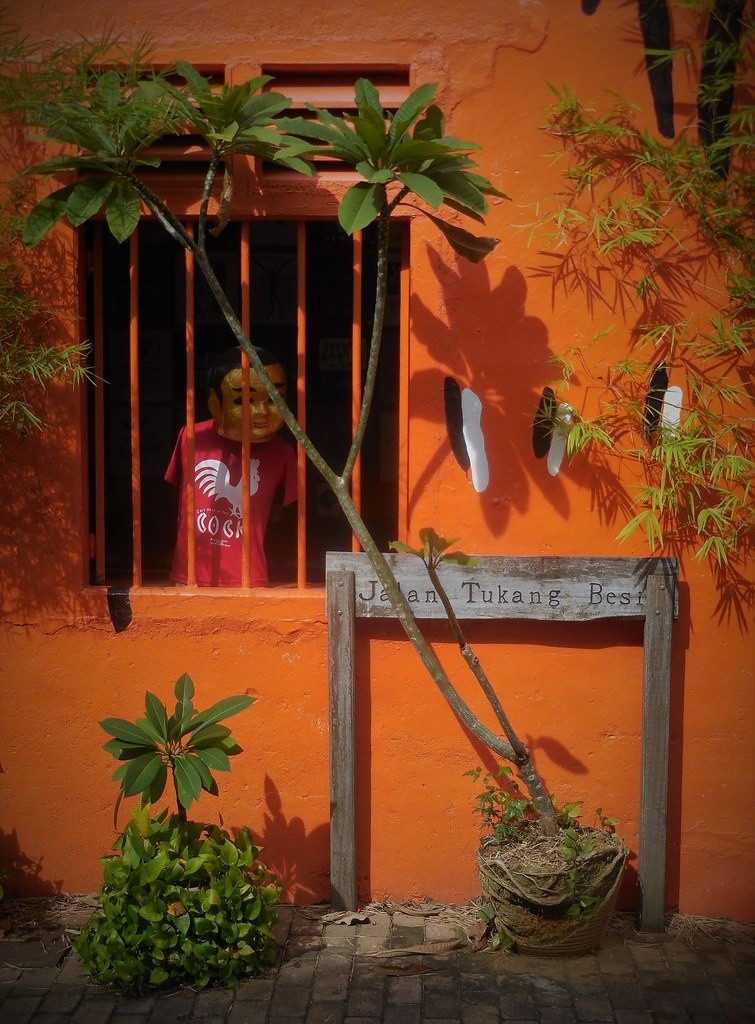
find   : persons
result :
[164,346,308,589]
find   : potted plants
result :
[20,64,631,955]
